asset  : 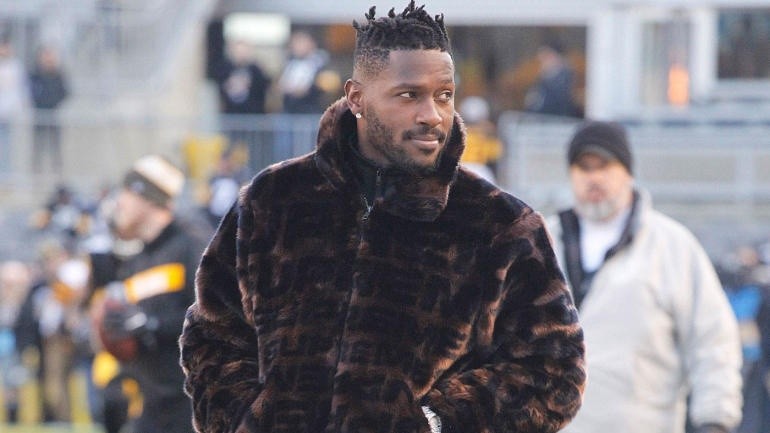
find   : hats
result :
[567,126,630,171]
[123,155,184,208]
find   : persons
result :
[208,37,267,114]
[714,236,770,372]
[279,28,330,113]
[7,156,214,433]
[180,0,586,433]
[206,170,240,231]
[0,29,32,170]
[522,35,578,120]
[26,42,67,186]
[734,296,770,433]
[544,121,743,433]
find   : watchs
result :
[421,406,442,433]
